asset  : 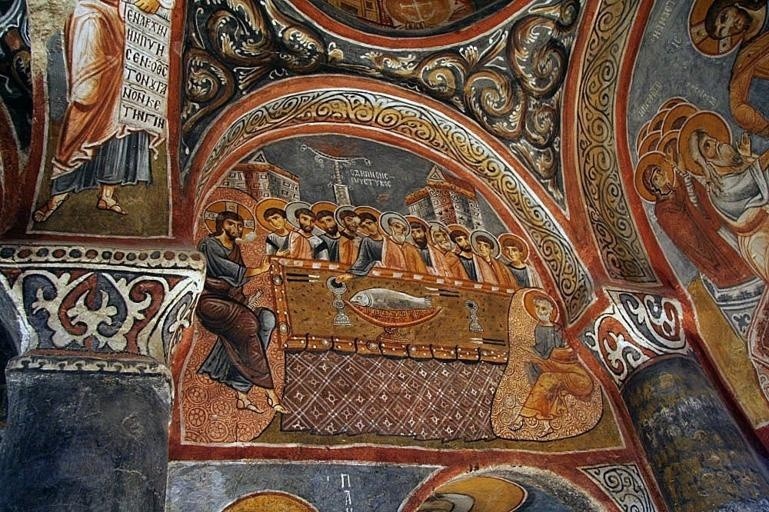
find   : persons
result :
[196,200,287,416]
[33,0,174,224]
[633,0,769,287]
[509,291,594,439]
[255,199,543,291]
